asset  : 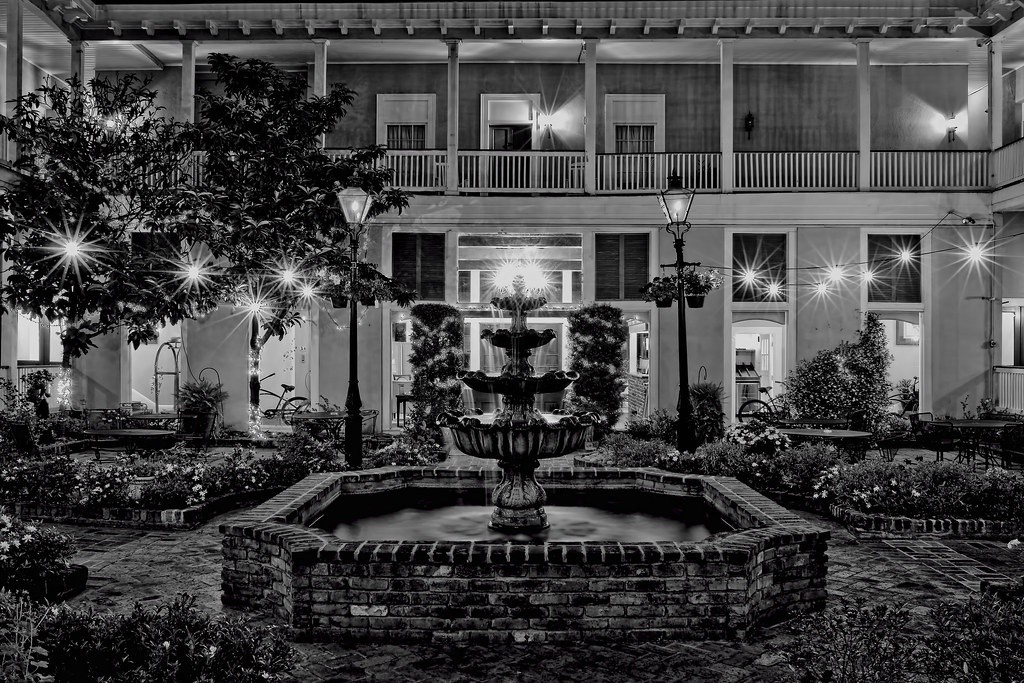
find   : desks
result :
[84,429,176,460]
[293,413,347,442]
[393,393,436,428]
[931,420,1018,474]
[778,426,869,463]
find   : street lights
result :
[333,168,377,468]
[653,170,705,452]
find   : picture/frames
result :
[896,320,918,345]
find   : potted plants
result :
[176,380,228,434]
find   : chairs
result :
[361,409,380,436]
[875,412,1024,475]
[173,407,219,458]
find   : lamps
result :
[744,110,756,141]
[947,114,960,141]
